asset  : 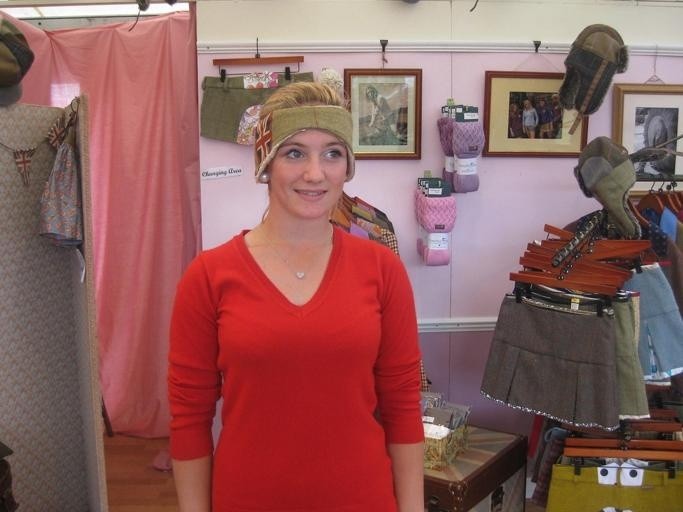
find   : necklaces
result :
[253,221,333,280]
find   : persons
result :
[508,93,562,139]
[363,84,406,145]
[166,80,426,510]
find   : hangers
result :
[212,38,304,82]
[667,174,682,210]
[601,198,649,228]
[510,223,651,317]
[635,174,664,217]
[676,191,683,204]
[563,385,683,478]
[656,174,680,216]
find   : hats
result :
[559,23,629,134]
[573,137,642,239]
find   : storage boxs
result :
[424,425,528,512]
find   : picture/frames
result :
[344,69,421,160]
[482,71,588,158]
[613,84,683,181]
